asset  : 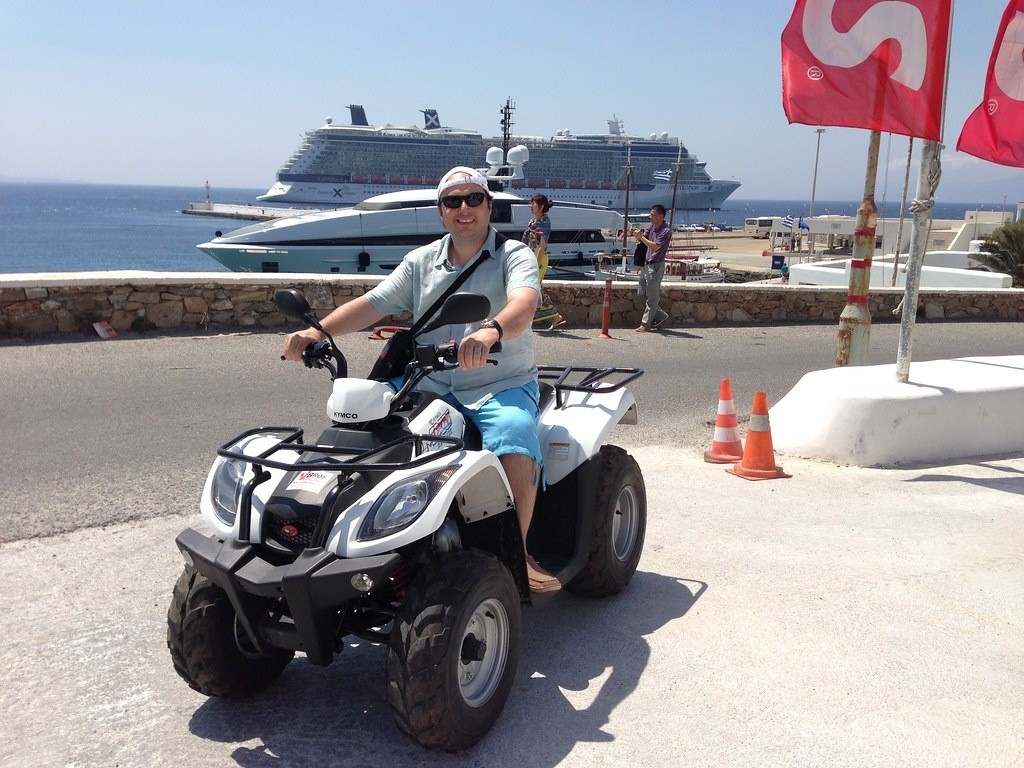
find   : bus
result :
[620,213,652,233]
[745,217,792,239]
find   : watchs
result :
[479,318,503,340]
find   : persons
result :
[284,166,561,594]
[634,204,673,332]
[781,263,789,281]
[791,237,796,252]
[521,195,567,332]
[796,233,801,246]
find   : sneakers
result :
[525,556,562,592]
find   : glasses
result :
[438,192,488,208]
[649,213,660,218]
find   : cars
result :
[676,222,733,233]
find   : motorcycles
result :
[166,288,648,756]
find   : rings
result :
[474,348,481,351]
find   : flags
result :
[653,169,674,182]
[956,0,1024,168]
[782,213,794,228]
[799,218,809,231]
[780,0,954,143]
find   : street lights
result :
[808,128,827,218]
[1001,193,1008,227]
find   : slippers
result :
[548,320,566,331]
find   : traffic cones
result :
[703,378,744,465]
[722,389,794,482]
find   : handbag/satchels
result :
[633,225,653,266]
[366,330,419,382]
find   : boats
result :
[528,179,546,188]
[570,181,583,189]
[195,95,642,274]
[390,177,405,185]
[425,178,440,185]
[549,180,567,188]
[601,182,612,189]
[353,175,367,184]
[371,176,385,184]
[585,181,599,189]
[552,136,729,284]
[618,183,630,190]
[511,180,525,187]
[407,177,423,185]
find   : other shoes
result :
[636,325,651,332]
[651,312,669,327]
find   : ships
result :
[256,105,743,212]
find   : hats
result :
[437,166,493,203]
